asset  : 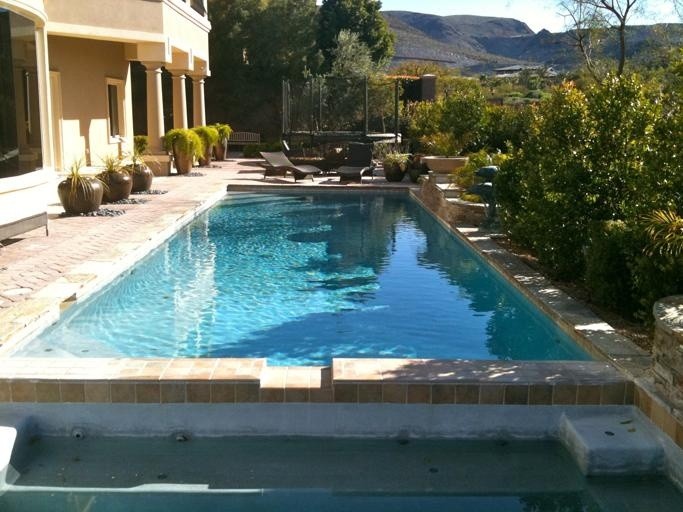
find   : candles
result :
[189,126,219,165]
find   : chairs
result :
[257,150,321,182]
[336,142,374,184]
[289,147,349,177]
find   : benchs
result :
[0,204,48,250]
[227,131,260,153]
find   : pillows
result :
[323,148,343,158]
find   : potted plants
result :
[207,122,233,160]
[160,127,203,175]
[380,138,410,181]
[120,134,154,193]
[422,131,470,173]
[55,157,106,213]
[408,154,429,183]
[94,154,133,203]
